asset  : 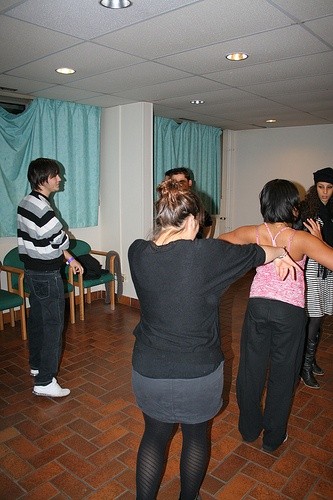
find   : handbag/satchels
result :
[75,253,109,280]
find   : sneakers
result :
[30,368,39,377]
[32,377,71,397]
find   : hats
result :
[313,167,333,183]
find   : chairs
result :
[0,239,123,341]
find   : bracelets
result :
[67,257,76,264]
[276,251,288,258]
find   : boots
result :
[299,337,320,389]
[312,357,325,375]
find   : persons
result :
[217,179,333,451]
[292,167,333,389]
[17,157,85,397]
[128,180,303,500]
[163,168,213,239]
